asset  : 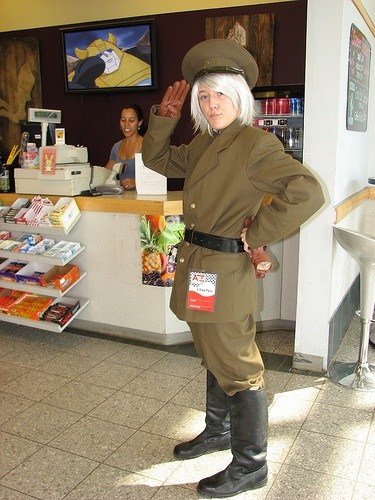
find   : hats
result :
[181,38,260,90]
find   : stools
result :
[328,225,375,391]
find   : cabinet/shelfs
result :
[0,213,89,333]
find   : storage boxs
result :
[0,196,81,328]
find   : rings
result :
[125,185,128,188]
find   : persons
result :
[142,38,325,498]
[105,103,145,190]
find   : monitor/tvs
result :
[17,119,56,148]
[59,18,159,95]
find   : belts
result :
[183,229,248,253]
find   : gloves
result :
[157,80,190,119]
[244,215,272,279]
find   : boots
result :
[174,369,234,460]
[196,384,269,497]
